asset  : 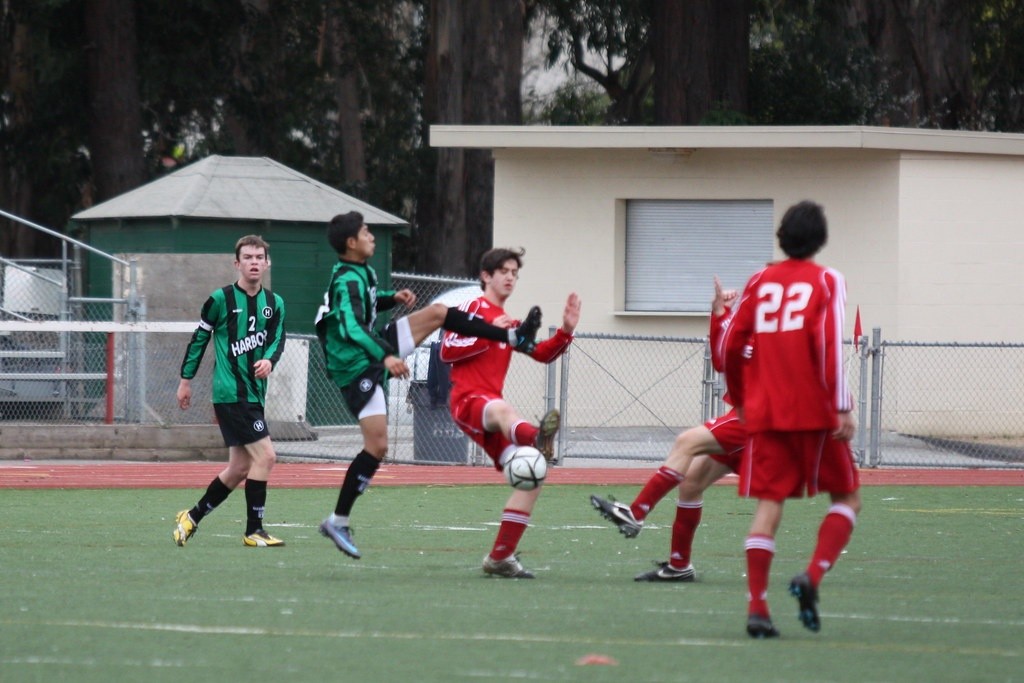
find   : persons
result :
[588,260,783,582]
[718,200,862,638]
[314,211,542,560]
[440,248,581,579]
[174,235,288,548]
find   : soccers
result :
[502,445,550,493]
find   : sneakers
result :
[789,572,820,632]
[242,529,285,547]
[591,493,644,538]
[634,560,696,581]
[747,614,780,638]
[535,410,561,461]
[516,306,541,353]
[174,510,198,547]
[483,551,535,578]
[319,513,361,559]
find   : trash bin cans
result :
[410,380,470,465]
